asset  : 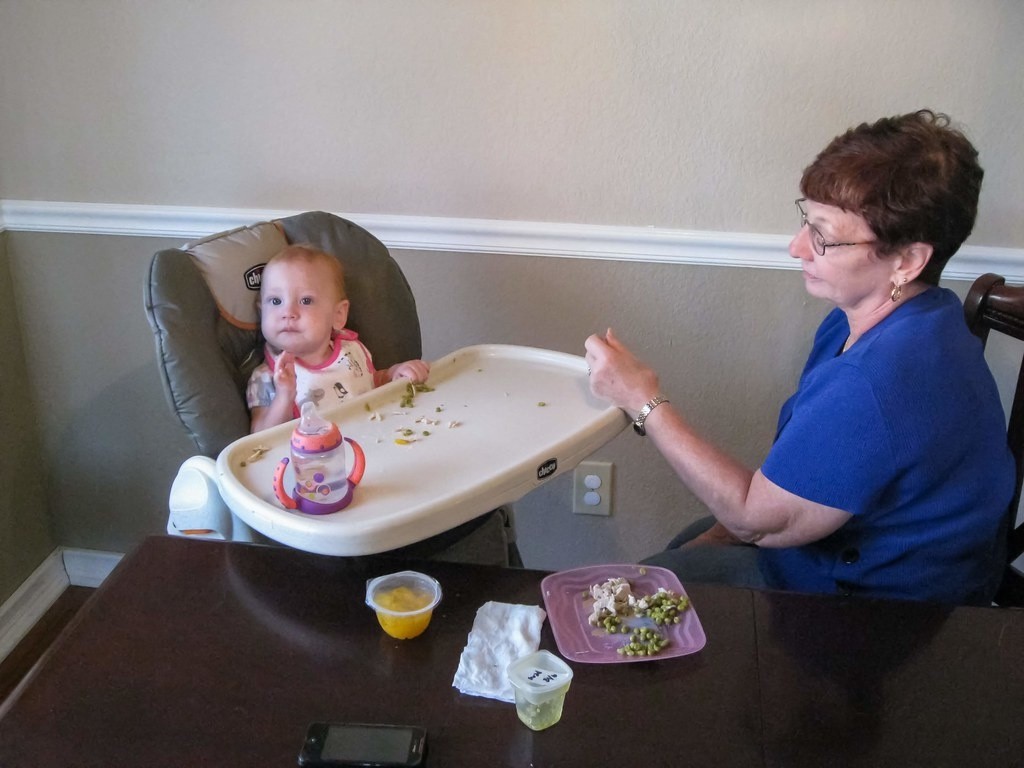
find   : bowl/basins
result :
[366,570,443,641]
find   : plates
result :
[540,564,706,664]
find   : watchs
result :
[632,395,669,436]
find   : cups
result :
[507,649,574,731]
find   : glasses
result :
[796,198,878,257]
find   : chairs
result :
[148,207,526,567]
[958,272,1024,611]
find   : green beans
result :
[583,567,688,656]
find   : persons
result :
[585,108,1016,603]
[246,244,430,436]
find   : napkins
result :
[453,599,547,703]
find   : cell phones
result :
[295,721,427,768]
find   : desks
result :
[0,535,1024,768]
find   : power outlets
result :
[571,457,614,519]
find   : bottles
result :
[273,400,366,516]
